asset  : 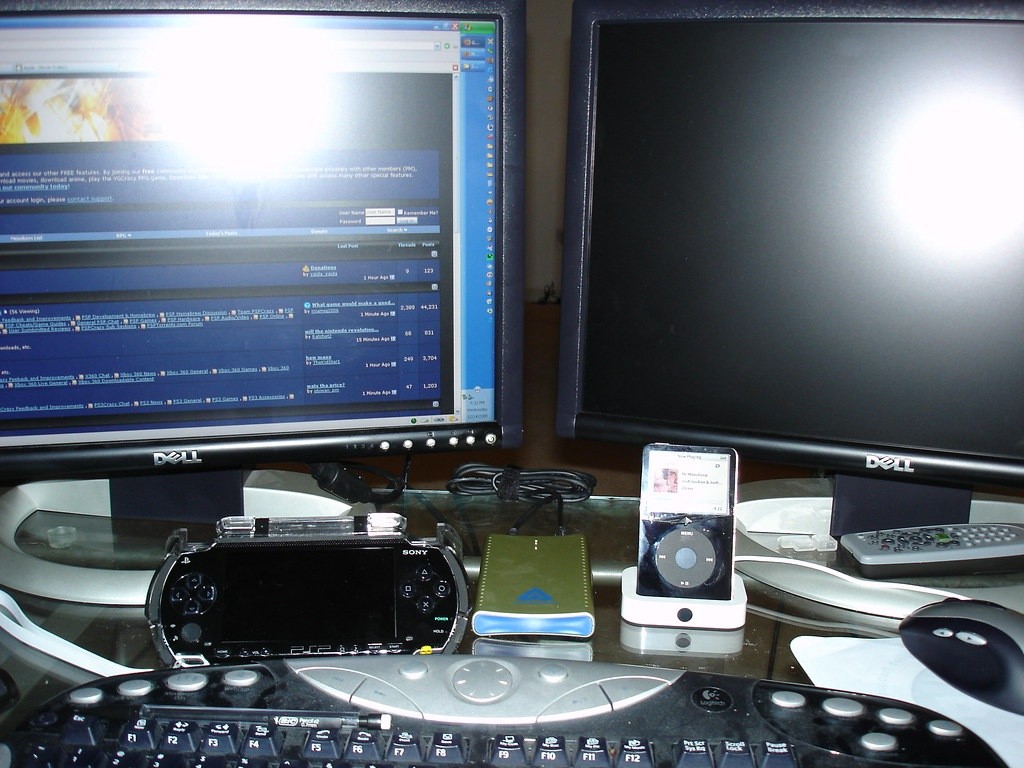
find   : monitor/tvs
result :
[0,0,521,603]
[551,0,1024,627]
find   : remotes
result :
[840,522,1024,579]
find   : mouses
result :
[899,598,1024,716]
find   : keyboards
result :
[0,649,1009,768]
